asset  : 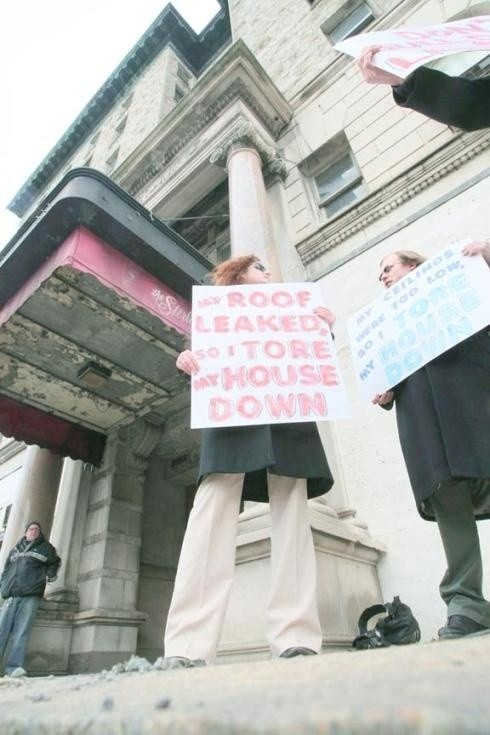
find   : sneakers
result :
[163,656,205,669]
[280,647,316,658]
[6,666,28,677]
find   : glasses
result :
[253,262,266,274]
[377,261,403,281]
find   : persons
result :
[372,240,490,638]
[358,44,490,131]
[0,522,61,677]
[156,254,336,669]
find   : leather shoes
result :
[437,615,489,638]
